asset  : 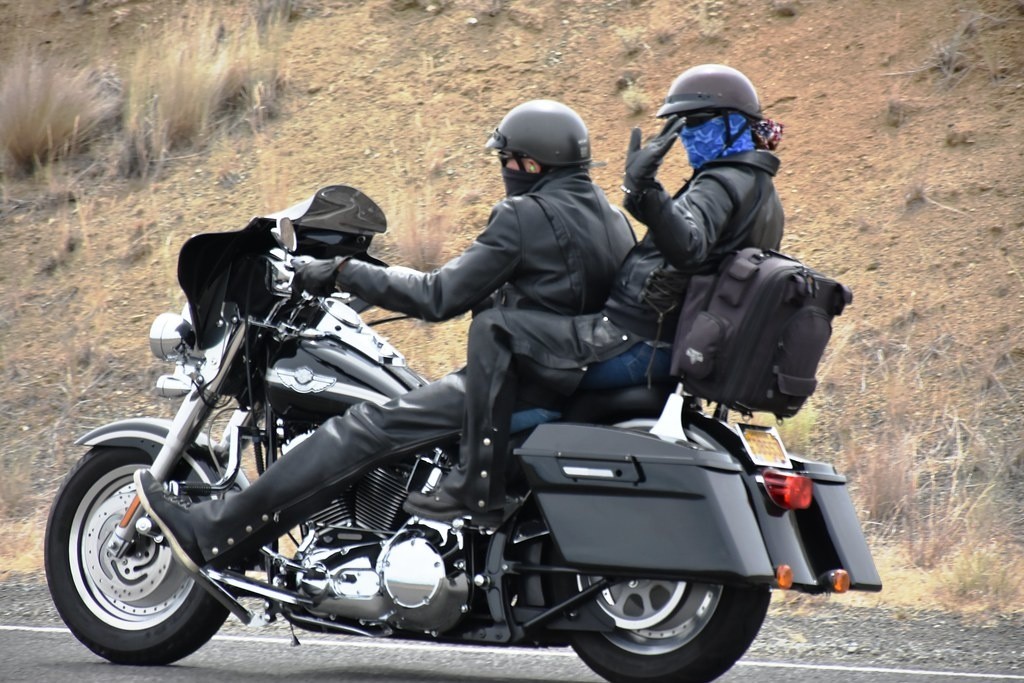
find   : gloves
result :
[289,255,349,303]
[618,114,687,192]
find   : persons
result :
[403,65,785,524]
[133,100,638,573]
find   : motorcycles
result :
[42,182,882,682]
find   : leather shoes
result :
[403,492,503,527]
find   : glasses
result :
[498,151,529,168]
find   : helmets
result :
[483,98,591,170]
[657,63,765,122]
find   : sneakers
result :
[134,468,205,572]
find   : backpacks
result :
[673,244,852,419]
